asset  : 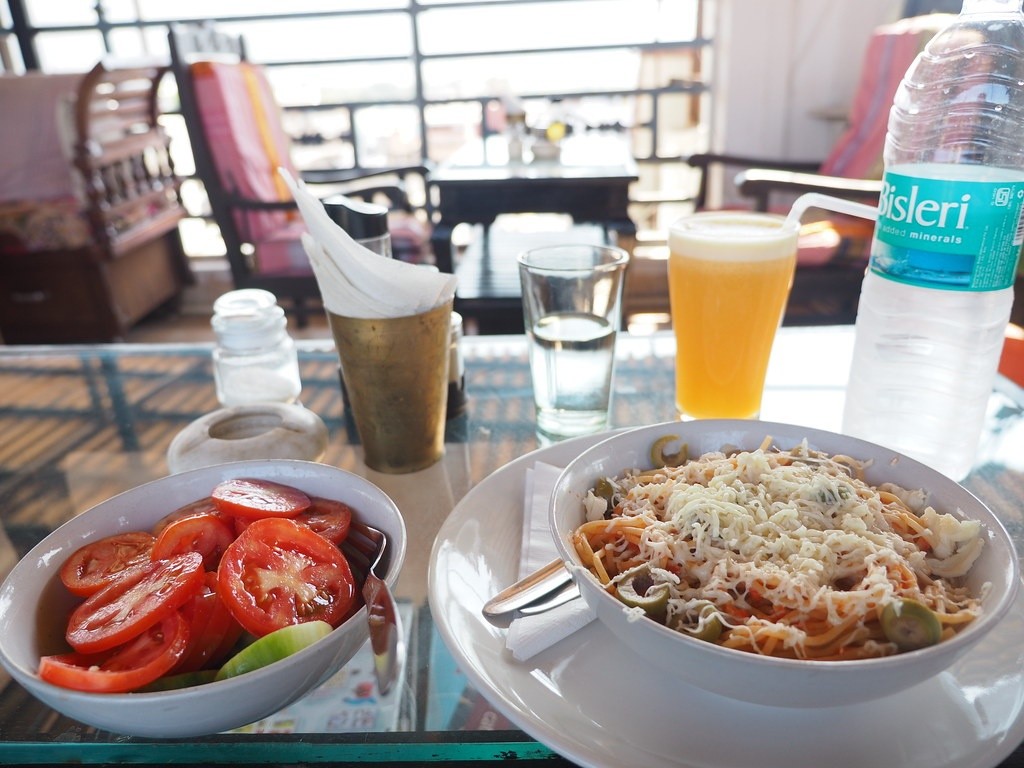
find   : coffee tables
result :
[426,128,639,333]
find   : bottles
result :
[839,1,1023,483]
[503,94,527,136]
[210,288,303,407]
[545,97,566,142]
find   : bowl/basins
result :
[0,458,409,740]
[546,420,1021,715]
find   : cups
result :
[322,298,454,475]
[665,211,803,420]
[516,242,631,448]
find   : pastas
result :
[570,434,980,662]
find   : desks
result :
[0,324,1023,767]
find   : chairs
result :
[174,56,432,328]
[685,14,1024,325]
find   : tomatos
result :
[35,477,357,697]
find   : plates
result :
[425,429,1023,768]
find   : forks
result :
[338,521,405,695]
